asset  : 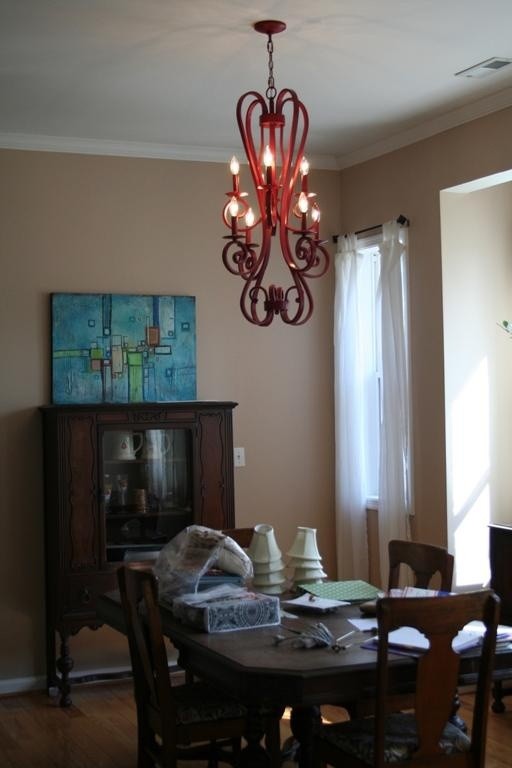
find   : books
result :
[358,586,511,658]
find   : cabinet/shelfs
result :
[38,400,239,708]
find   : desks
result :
[155,577,512,766]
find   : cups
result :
[109,429,171,462]
[248,523,328,596]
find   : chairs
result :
[386,539,456,594]
[124,561,259,765]
[314,590,500,768]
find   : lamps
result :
[220,19,330,325]
